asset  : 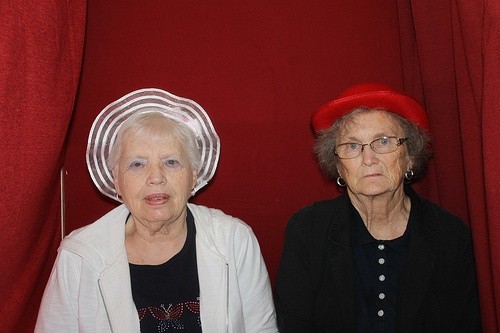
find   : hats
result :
[85,88,221,202]
[311,82,429,136]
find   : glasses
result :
[334,136,410,159]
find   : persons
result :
[274,84,481,333]
[34,88,279,333]
